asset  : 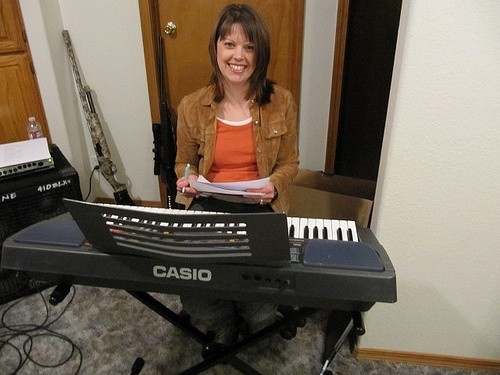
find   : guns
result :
[62,30,137,208]
[151,35,188,210]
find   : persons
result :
[170,4,301,215]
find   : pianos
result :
[1,199,400,312]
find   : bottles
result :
[26,117,43,139]
